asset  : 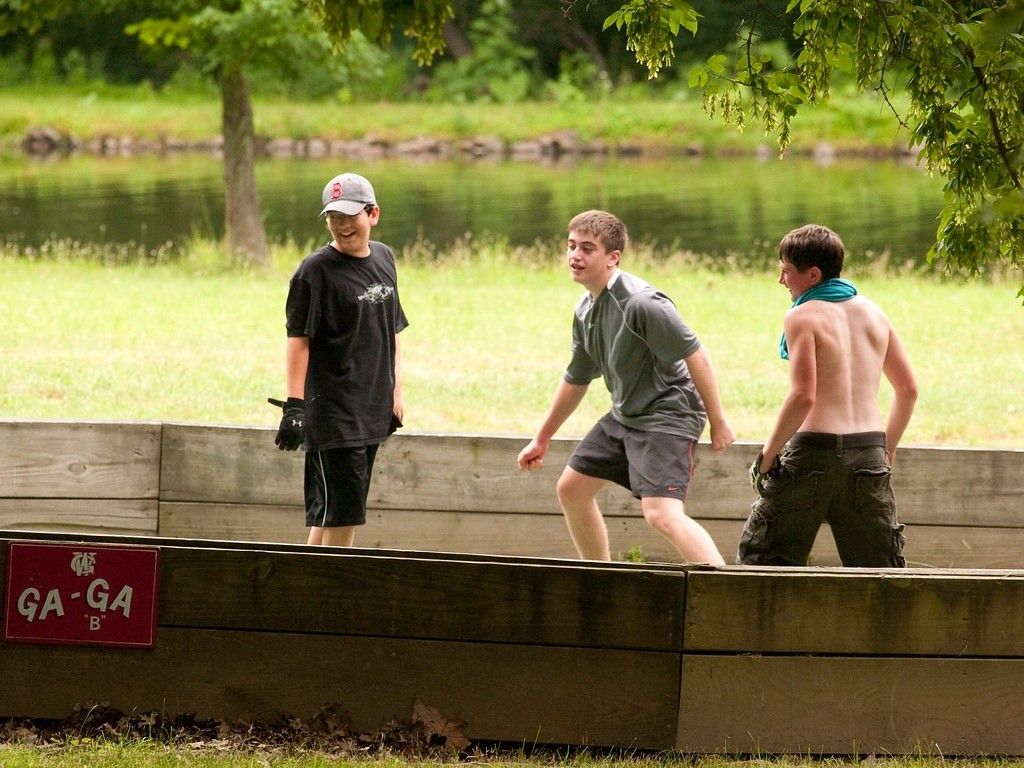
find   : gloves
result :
[749,453,781,498]
[268,397,306,451]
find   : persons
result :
[275,173,408,547]
[736,224,919,569]
[516,210,736,565]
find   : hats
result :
[318,173,376,216]
